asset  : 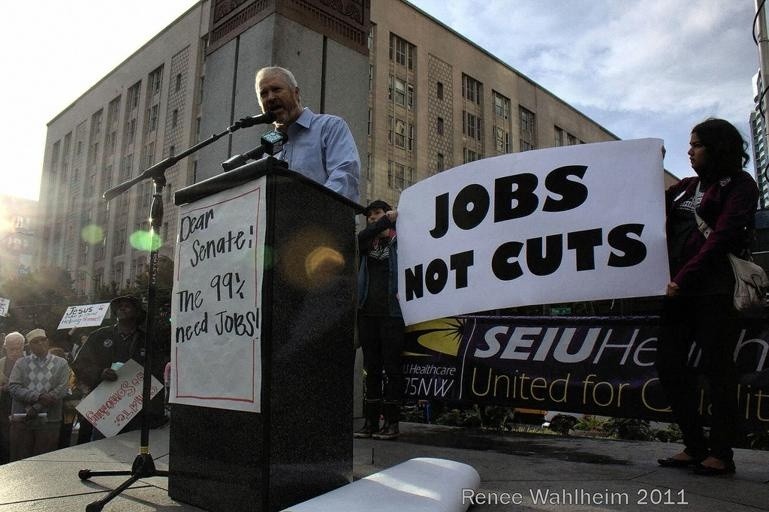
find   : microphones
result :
[244,126,288,161]
[235,110,277,129]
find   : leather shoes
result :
[658,457,736,475]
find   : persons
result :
[243,65,359,204]
[0,295,171,465]
[656,116,760,477]
[351,198,407,441]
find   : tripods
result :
[79,123,242,512]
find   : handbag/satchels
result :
[727,253,769,313]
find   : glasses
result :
[30,337,47,344]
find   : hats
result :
[110,294,146,326]
[26,328,47,345]
[363,200,391,217]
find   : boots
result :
[354,398,401,439]
